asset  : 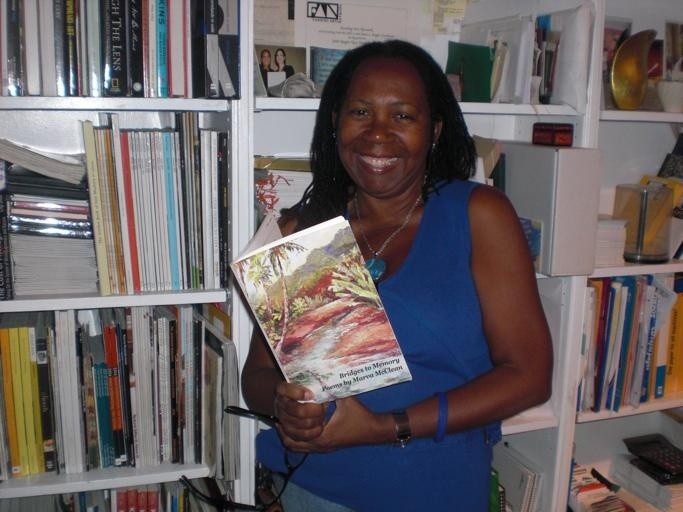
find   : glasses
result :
[180,406,311,512]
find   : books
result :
[444,0,682,277]
[1,305,234,512]
[490,274,682,511]
[1,0,241,100]
[1,111,234,302]
[226,209,416,405]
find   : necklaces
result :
[350,185,426,286]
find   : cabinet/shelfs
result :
[0,0,683,512]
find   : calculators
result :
[622,433,683,475]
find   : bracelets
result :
[388,404,410,448]
[432,388,449,445]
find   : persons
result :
[273,47,294,80]
[256,47,274,91]
[236,38,554,509]
